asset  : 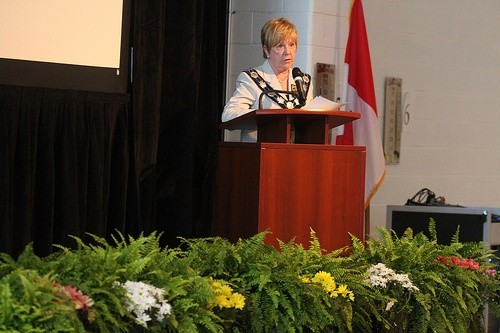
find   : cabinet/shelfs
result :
[386,204,500,333]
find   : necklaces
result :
[276,76,289,83]
[270,59,289,76]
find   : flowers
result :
[0,217,500,333]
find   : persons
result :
[221,17,313,143]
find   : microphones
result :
[292,67,307,106]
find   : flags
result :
[331,1,386,210]
